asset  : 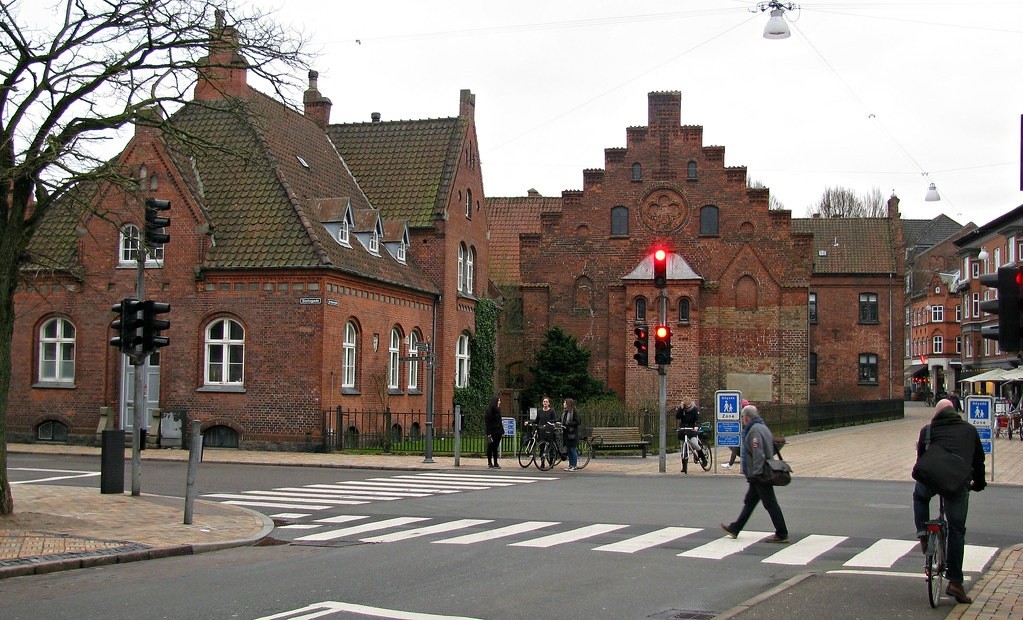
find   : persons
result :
[547,398,580,471]
[719,404,794,543]
[484,396,505,469]
[524,397,557,470]
[719,398,749,468]
[673,396,709,472]
[924,386,963,413]
[911,397,988,604]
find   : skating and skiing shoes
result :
[945,582,972,603]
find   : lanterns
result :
[912,377,928,383]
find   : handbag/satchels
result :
[912,444,974,502]
[763,456,792,486]
[542,428,556,442]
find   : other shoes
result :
[494,464,501,468]
[570,467,578,472]
[565,467,571,471]
[487,464,493,468]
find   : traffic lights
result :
[633,325,649,366]
[653,245,666,289]
[142,299,171,354]
[110,298,123,352]
[122,298,142,356]
[144,197,171,244]
[655,325,670,365]
[979,265,1023,351]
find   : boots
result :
[697,449,707,466]
[681,459,686,472]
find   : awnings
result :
[904,363,928,379]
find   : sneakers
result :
[766,535,790,542]
[721,462,732,469]
[721,522,737,538]
[921,540,928,554]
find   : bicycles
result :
[673,427,712,474]
[994,411,1006,439]
[922,484,985,609]
[1004,412,1016,440]
[518,421,592,471]
[924,393,946,407]
[1017,410,1023,441]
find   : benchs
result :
[586,426,653,458]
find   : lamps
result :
[925,183,940,201]
[763,0,791,40]
[978,246,989,259]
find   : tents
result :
[957,365,1023,397]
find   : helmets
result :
[742,399,749,407]
